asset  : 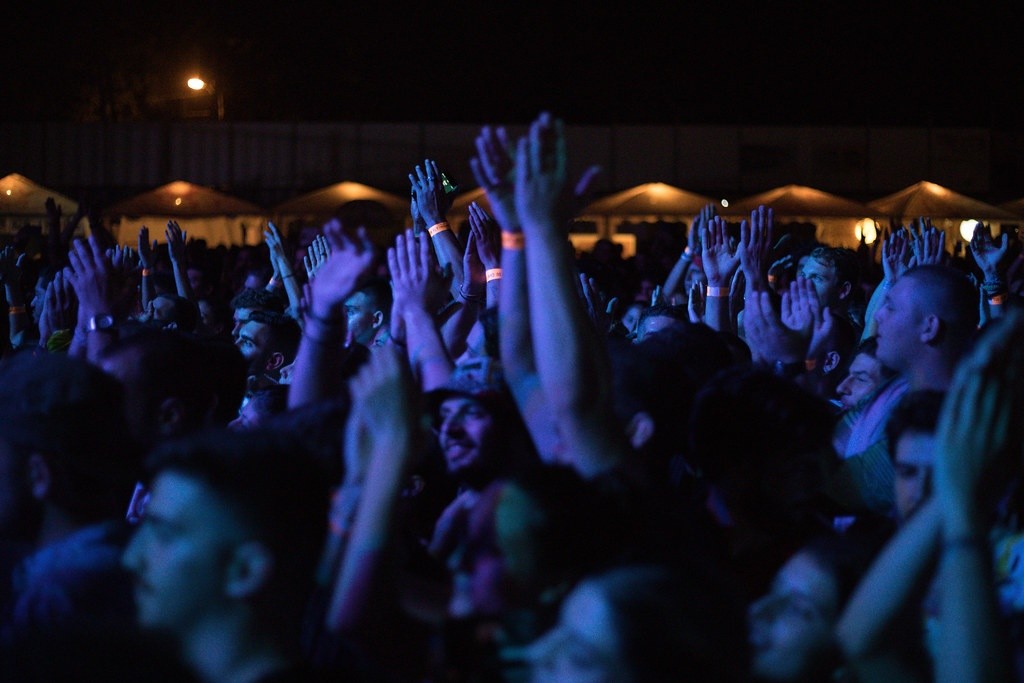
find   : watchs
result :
[87,313,113,333]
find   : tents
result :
[272,181,410,236]
[0,172,78,234]
[102,181,275,248]
[997,199,1024,240]
[570,181,725,258]
[868,181,1024,256]
[714,184,889,259]
[445,188,493,237]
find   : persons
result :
[0,112,1024,683]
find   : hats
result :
[423,354,511,412]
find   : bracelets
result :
[9,305,26,314]
[943,534,995,561]
[484,268,503,282]
[502,231,525,250]
[427,221,448,238]
[281,273,294,279]
[459,285,472,301]
[142,268,154,277]
[298,312,331,347]
[74,328,87,347]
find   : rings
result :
[427,175,436,181]
[319,252,326,255]
